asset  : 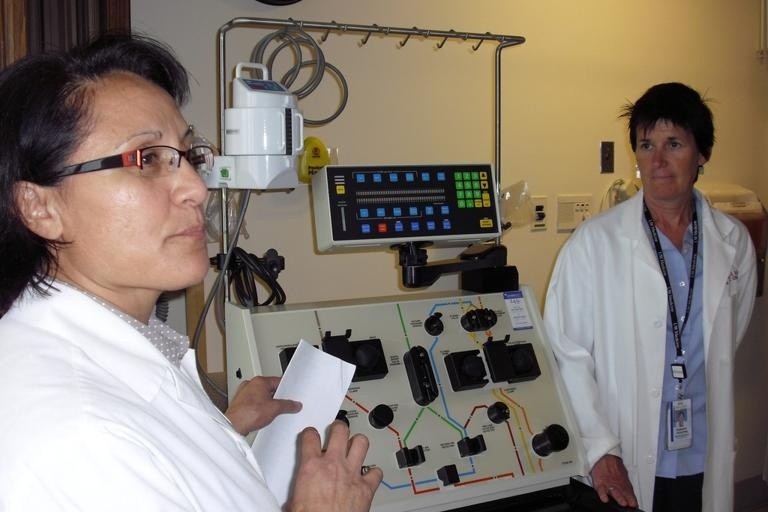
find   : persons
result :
[542,82,759,512]
[0,36,382,512]
[674,412,686,428]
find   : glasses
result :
[50,145,214,178]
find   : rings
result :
[607,486,614,495]
